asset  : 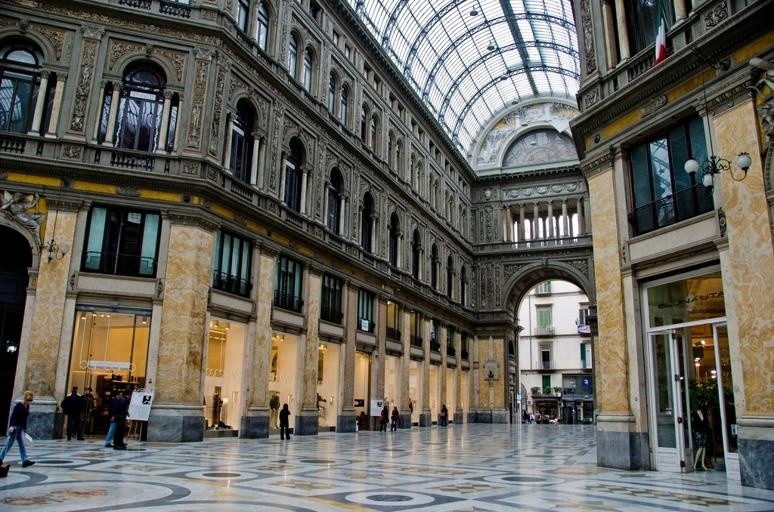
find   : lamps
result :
[683,40,752,199]
[470,4,538,148]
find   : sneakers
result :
[67,434,86,441]
[104,442,127,450]
[22,460,35,468]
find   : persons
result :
[210,393,223,428]
[278,403,291,441]
[269,391,280,429]
[440,405,447,427]
[357,411,368,430]
[692,395,715,470]
[0,391,36,468]
[102,389,132,451]
[408,398,413,413]
[379,406,388,432]
[60,386,94,441]
[534,411,542,420]
[390,407,398,432]
